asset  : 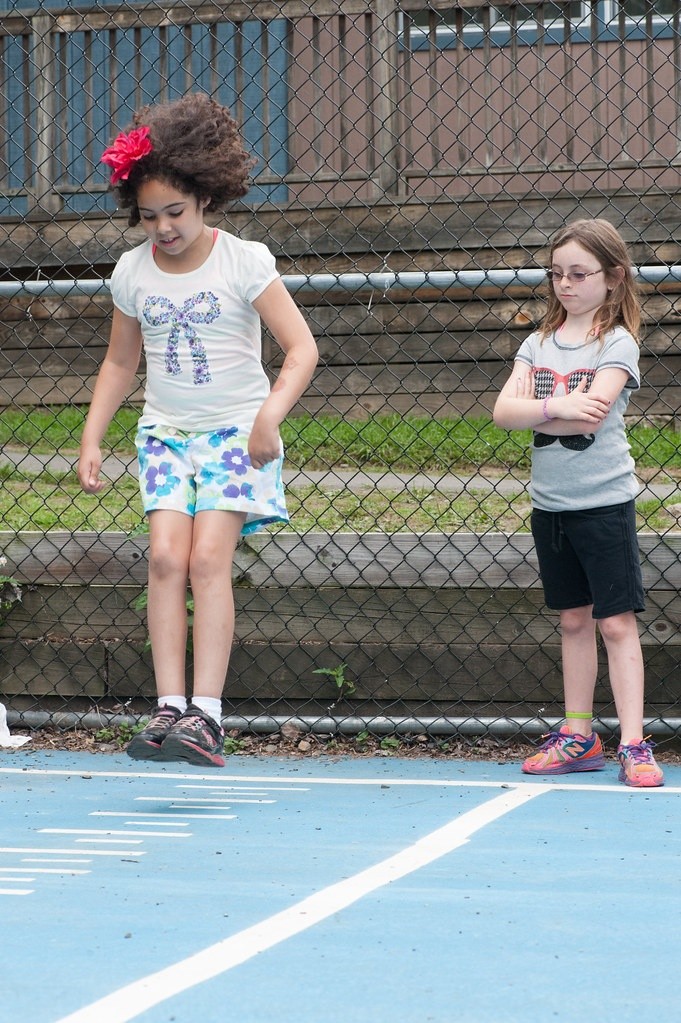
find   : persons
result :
[79,92,318,770]
[493,219,665,788]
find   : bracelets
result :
[542,397,551,420]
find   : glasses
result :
[547,269,604,283]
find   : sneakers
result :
[521,721,606,775]
[160,704,225,767]
[126,706,182,760]
[617,734,664,787]
